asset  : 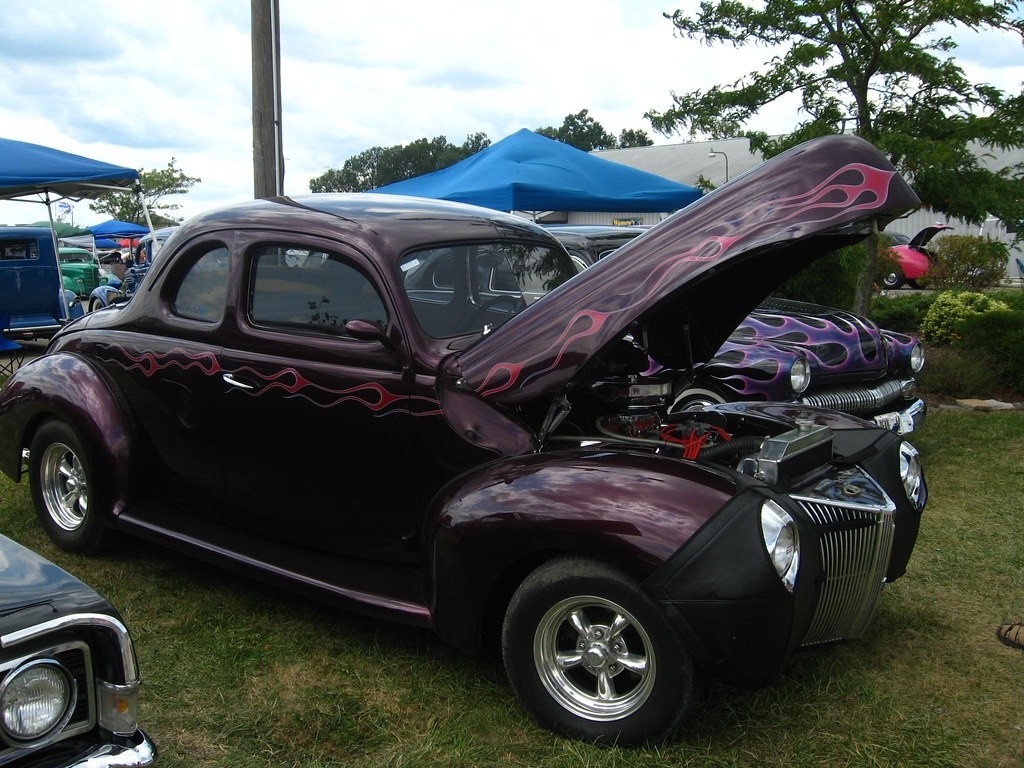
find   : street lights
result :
[707,147,728,183]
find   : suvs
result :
[0,134,930,748]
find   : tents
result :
[349,129,705,218]
[0,135,160,325]
[59,219,151,277]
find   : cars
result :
[87,223,179,316]
[299,222,660,339]
[1,535,160,768]
[0,225,119,355]
[876,221,956,291]
